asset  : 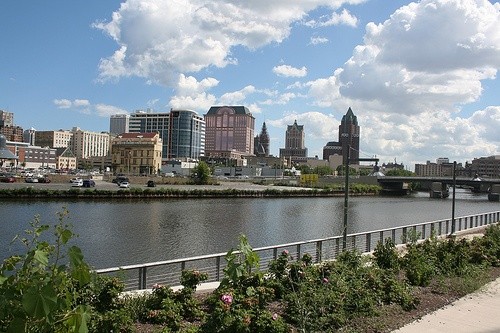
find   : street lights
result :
[450,160,481,234]
[342,145,385,251]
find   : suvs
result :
[113,177,129,183]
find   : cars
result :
[120,182,130,188]
[83,179,96,187]
[0,173,16,183]
[26,177,39,183]
[39,178,51,183]
[70,177,83,183]
[72,181,83,187]
[148,181,157,187]
